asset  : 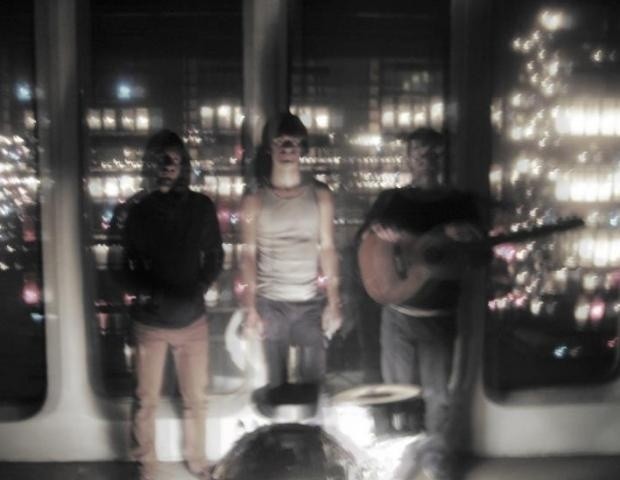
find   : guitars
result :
[358,214,584,303]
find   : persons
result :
[354,127,477,480]
[108,129,225,480]
[235,114,343,421]
